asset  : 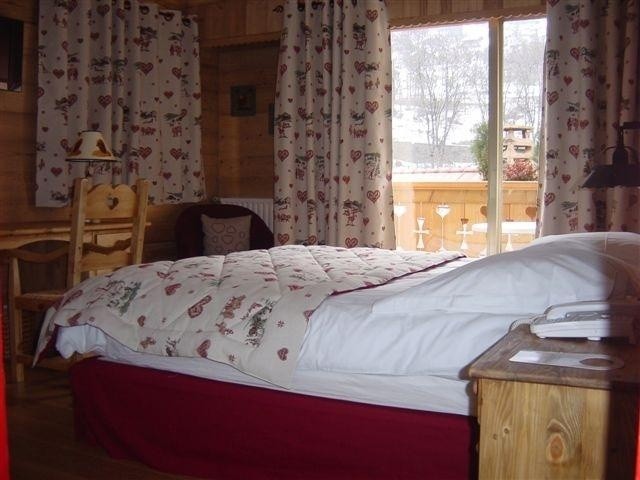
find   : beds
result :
[55,243,640,480]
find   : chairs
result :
[9,179,150,381]
[174,205,274,258]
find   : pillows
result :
[200,212,252,257]
[373,232,640,316]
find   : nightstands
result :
[466,322,640,479]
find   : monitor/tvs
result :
[0,15,24,92]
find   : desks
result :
[0,221,152,382]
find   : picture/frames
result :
[230,84,273,135]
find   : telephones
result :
[529,299,639,342]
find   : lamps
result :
[581,118,640,189]
[65,131,117,178]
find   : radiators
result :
[213,197,274,235]
